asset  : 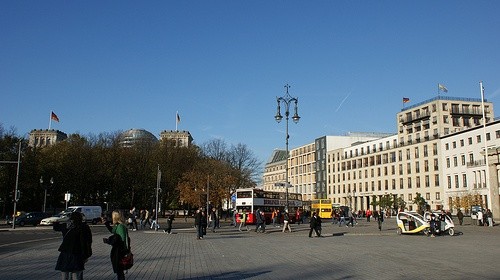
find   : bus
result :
[234,186,304,226]
[310,198,333,219]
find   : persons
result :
[195,207,207,239]
[208,207,221,230]
[101,210,127,280]
[296,210,311,224]
[472,208,492,226]
[308,212,322,237]
[375,211,383,231]
[271,209,282,226]
[238,209,251,231]
[446,210,451,218]
[457,210,464,226]
[127,207,160,231]
[255,208,266,233]
[52,211,93,280]
[366,210,371,222]
[331,208,358,228]
[282,213,292,232]
[162,211,175,234]
[425,214,436,237]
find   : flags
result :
[439,84,448,93]
[177,115,181,121]
[51,112,59,122]
[403,98,409,103]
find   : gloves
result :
[105,222,113,232]
[103,238,109,243]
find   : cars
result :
[39,212,70,226]
[7,211,47,226]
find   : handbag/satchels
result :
[119,250,133,269]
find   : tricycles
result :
[396,211,430,237]
[423,209,456,236]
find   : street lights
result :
[274,84,301,232]
[40,175,54,212]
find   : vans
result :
[63,205,103,225]
